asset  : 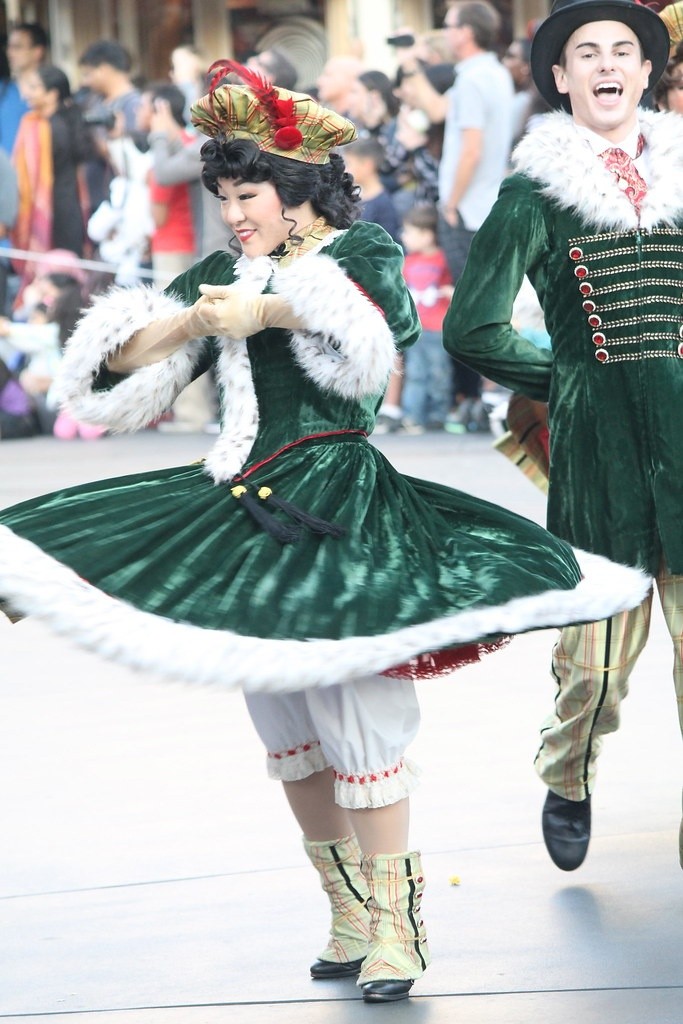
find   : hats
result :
[189,59,358,164]
[530,0,671,116]
[35,249,91,307]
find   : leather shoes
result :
[542,788,591,871]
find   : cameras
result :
[82,106,115,129]
[386,35,416,49]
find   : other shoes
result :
[372,405,402,436]
[397,418,423,437]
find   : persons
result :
[0,56,653,1001]
[0,0,683,440]
[443,0,683,872]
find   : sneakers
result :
[442,397,495,435]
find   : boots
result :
[356,850,432,1001]
[302,832,373,978]
